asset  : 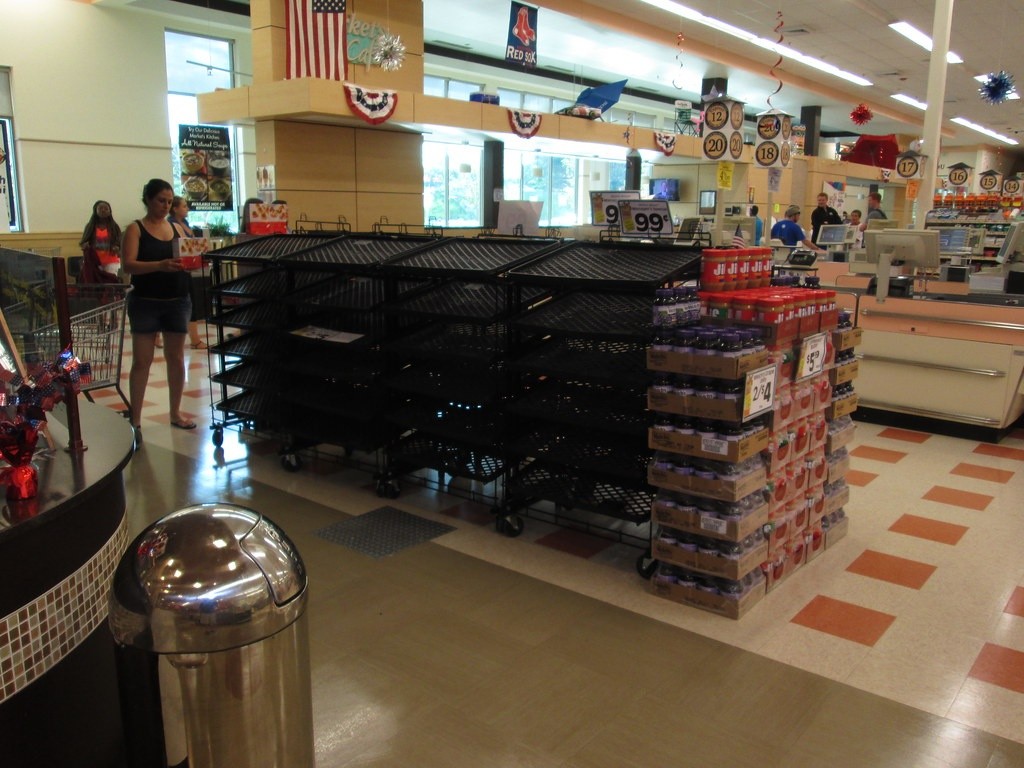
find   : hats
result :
[784,204,801,215]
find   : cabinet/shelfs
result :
[202,232,709,580]
[923,200,1024,272]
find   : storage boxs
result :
[647,329,861,623]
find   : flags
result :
[732,226,744,249]
[284,0,348,81]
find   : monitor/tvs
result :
[675,215,704,245]
[816,219,986,269]
[649,178,680,201]
[722,216,756,246]
[997,221,1024,265]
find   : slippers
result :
[132,424,142,449]
[172,418,197,430]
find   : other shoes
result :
[107,321,119,333]
[96,322,106,335]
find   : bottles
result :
[650,245,856,599]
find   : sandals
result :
[190,340,208,350]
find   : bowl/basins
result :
[183,153,231,200]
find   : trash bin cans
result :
[108,503,315,768]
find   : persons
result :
[81,200,120,333]
[155,196,207,349]
[859,193,886,231]
[771,205,821,250]
[810,193,842,250]
[849,209,863,248]
[240,198,291,233]
[121,179,197,447]
[749,205,762,246]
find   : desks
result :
[0,389,134,540]
